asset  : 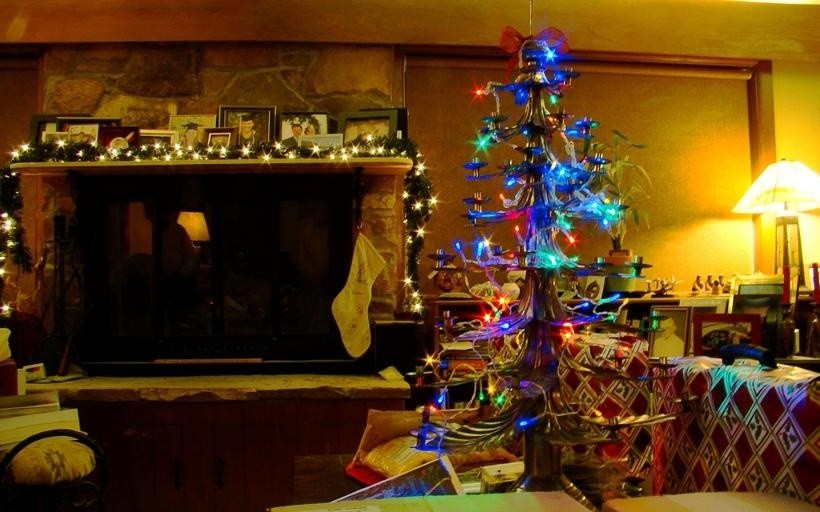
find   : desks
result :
[421,297,820,410]
[270,490,592,512]
[601,491,820,512]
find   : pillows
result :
[352,408,480,468]
[360,435,516,479]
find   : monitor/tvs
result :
[55,170,372,377]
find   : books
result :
[732,272,783,296]
[442,339,494,372]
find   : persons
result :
[692,274,705,292]
[718,274,726,287]
[651,315,685,358]
[283,124,304,150]
[300,123,323,150]
[50,132,96,144]
[237,115,264,158]
[178,127,204,155]
[704,275,715,291]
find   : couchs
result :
[496,330,650,487]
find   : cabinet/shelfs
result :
[61,399,406,512]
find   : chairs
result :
[0,429,109,512]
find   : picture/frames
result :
[342,115,391,149]
[135,128,179,155]
[167,113,217,150]
[648,274,796,364]
[201,127,240,153]
[96,125,140,154]
[217,106,279,149]
[40,130,72,150]
[54,116,122,131]
[277,111,331,151]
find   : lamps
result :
[175,211,211,244]
[730,158,820,297]
